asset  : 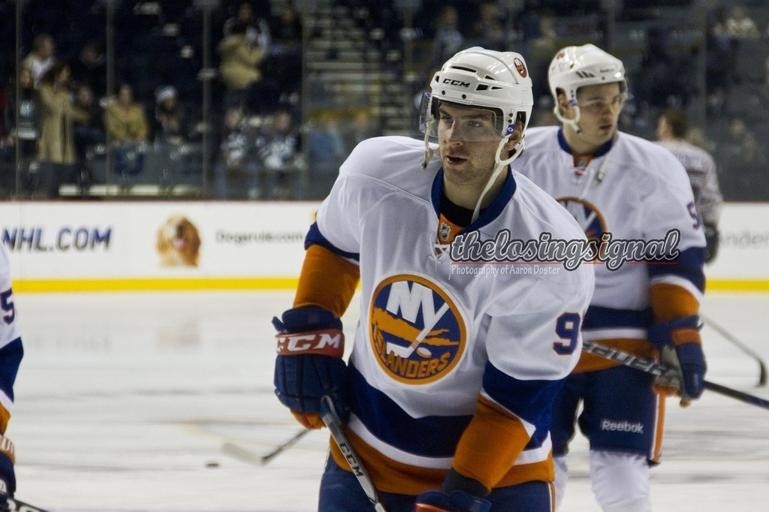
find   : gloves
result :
[702,220,722,265]
[270,303,352,429]
[643,314,708,408]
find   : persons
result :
[648,109,723,268]
[382,1,768,201]
[1,1,310,203]
[0,245,26,512]
[266,44,603,512]
[511,44,712,512]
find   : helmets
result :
[418,45,534,168]
[548,41,629,133]
